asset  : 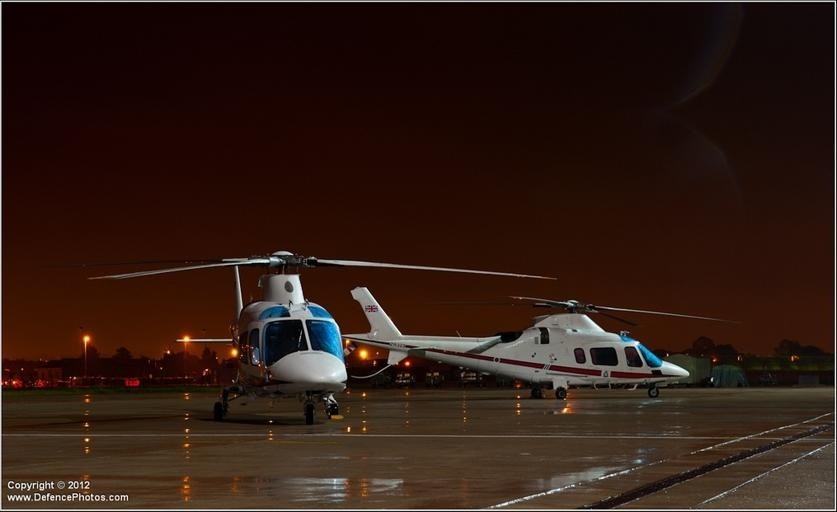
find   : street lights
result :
[83,335,90,360]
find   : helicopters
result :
[88,250,557,424]
[342,286,741,400]
